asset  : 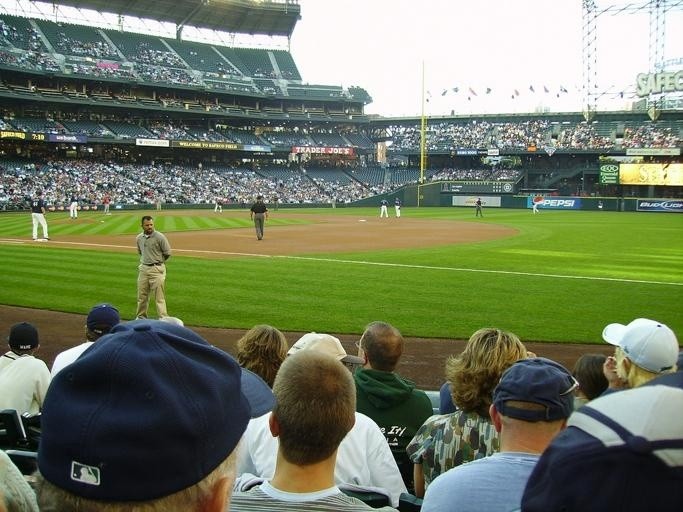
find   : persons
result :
[405,318,682,512]
[250,195,268,240]
[136,216,172,320]
[2,305,433,511]
[30,189,51,240]
[1,19,683,219]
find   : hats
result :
[37,319,278,502]
[493,357,575,421]
[285,330,365,364]
[9,322,37,351]
[600,319,678,374]
[87,305,121,333]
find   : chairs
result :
[1,14,683,196]
[1,407,423,511]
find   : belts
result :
[144,263,160,267]
